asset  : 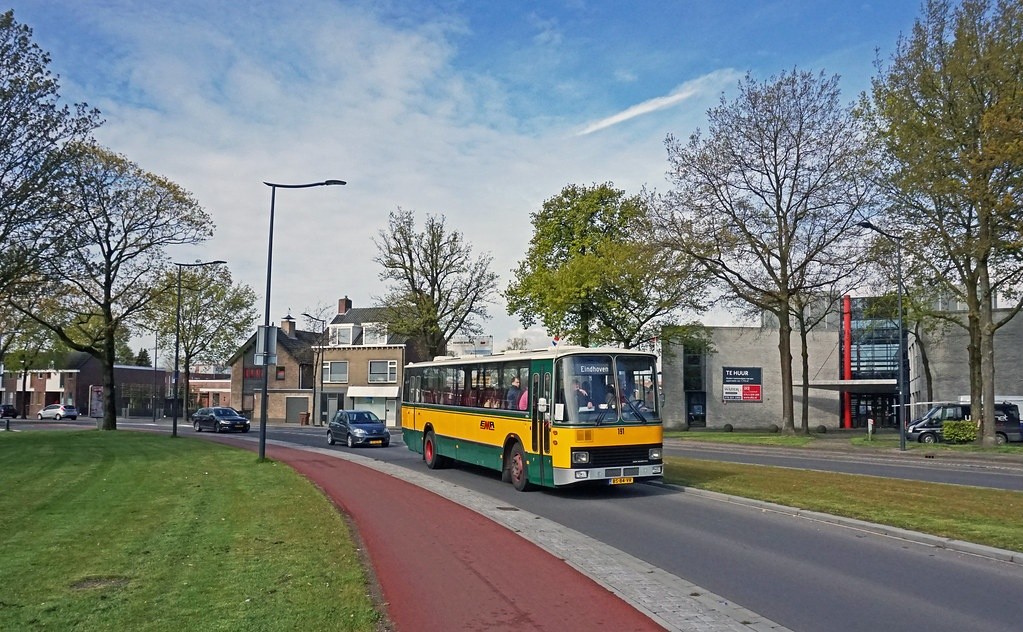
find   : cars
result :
[0,404,19,419]
[327,410,390,448]
[191,407,250,434]
[37,404,77,421]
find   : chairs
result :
[410,386,508,409]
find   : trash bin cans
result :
[299,412,310,426]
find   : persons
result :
[573,380,592,409]
[506,377,522,410]
[517,386,529,411]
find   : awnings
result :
[346,386,401,398]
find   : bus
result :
[401,345,664,492]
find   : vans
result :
[905,403,1021,445]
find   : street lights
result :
[259,179,347,462]
[857,222,906,450]
[301,313,324,427]
[173,260,227,436]
[137,324,159,422]
